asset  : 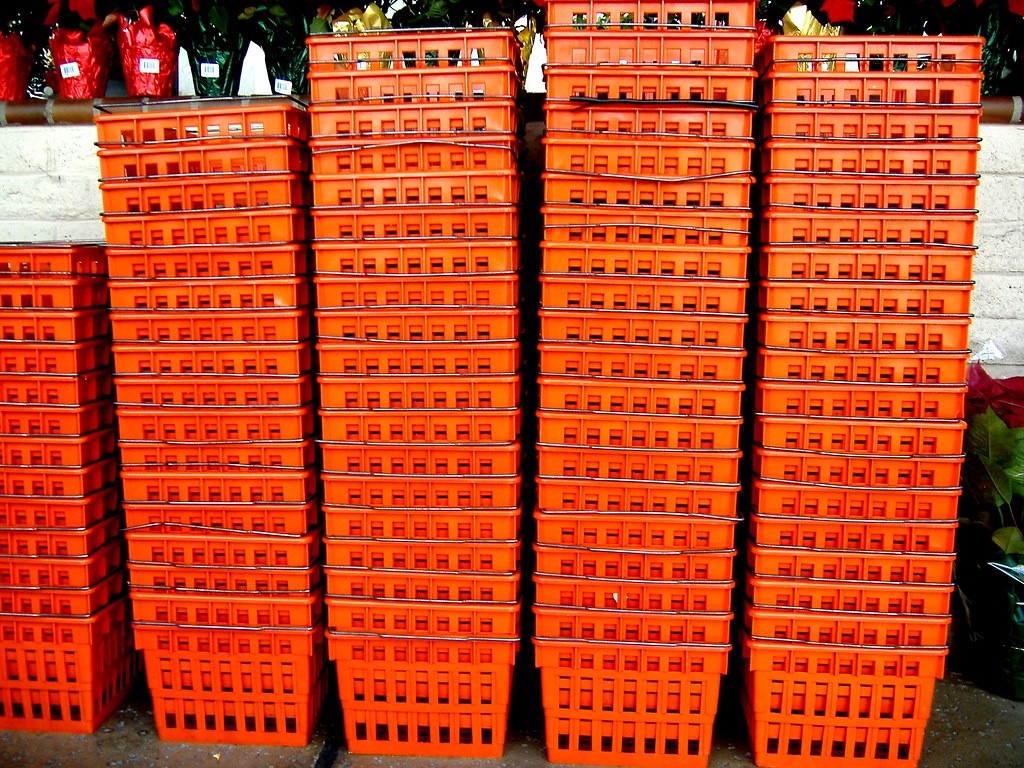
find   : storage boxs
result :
[0,0,986,768]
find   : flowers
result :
[959,359,1024,700]
